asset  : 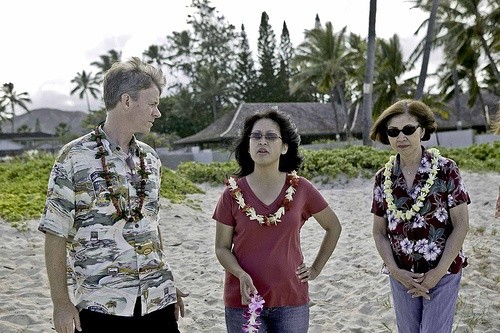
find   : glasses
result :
[249,132,283,141]
[387,124,421,138]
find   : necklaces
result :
[93,123,148,224]
[225,168,300,227]
[383,147,441,222]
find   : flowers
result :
[225,170,299,226]
[384,147,441,221]
[241,292,265,332]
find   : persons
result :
[209,104,344,333]
[367,98,473,333]
[36,55,193,333]
